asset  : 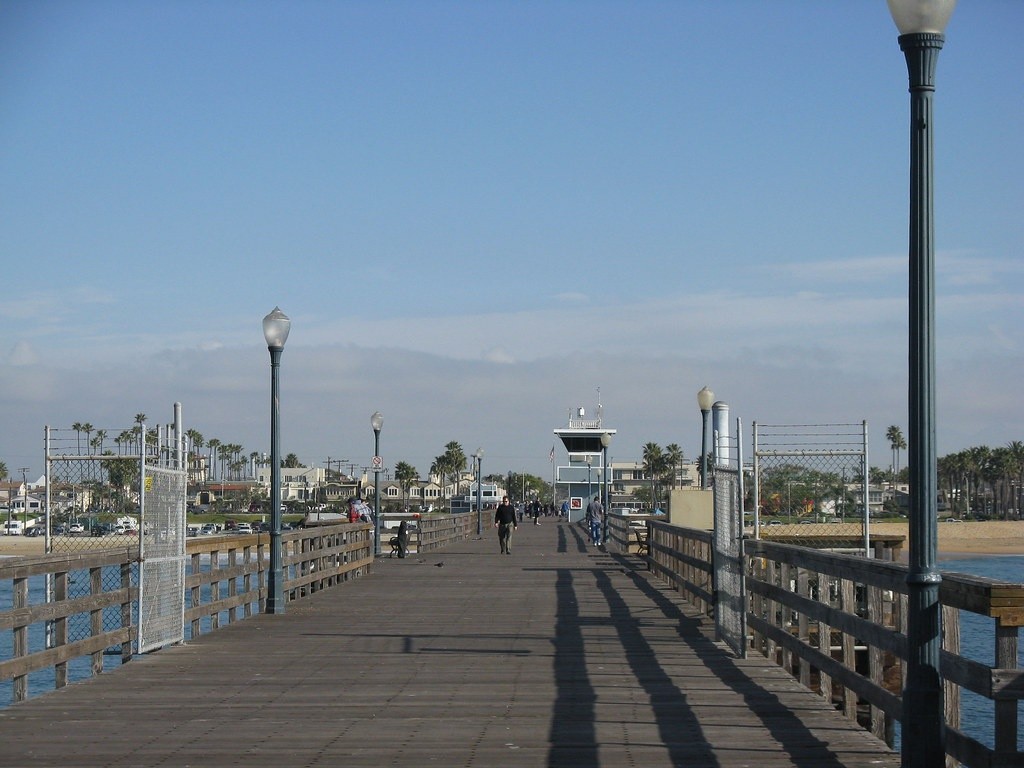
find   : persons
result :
[515,500,569,526]
[348,498,375,525]
[494,496,518,554]
[587,497,605,546]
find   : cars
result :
[115,525,126,535]
[186,521,292,537]
[281,506,287,513]
[23,526,45,537]
[52,525,68,535]
[187,505,208,514]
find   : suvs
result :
[249,503,263,512]
[69,523,84,534]
[91,522,111,537]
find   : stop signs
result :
[373,457,382,465]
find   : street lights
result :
[471,455,477,480]
[370,411,385,555]
[587,454,593,504]
[600,431,612,543]
[476,447,485,534]
[888,1,956,768]
[596,469,602,504]
[261,306,291,615]
[697,384,715,490]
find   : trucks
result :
[3,521,22,535]
